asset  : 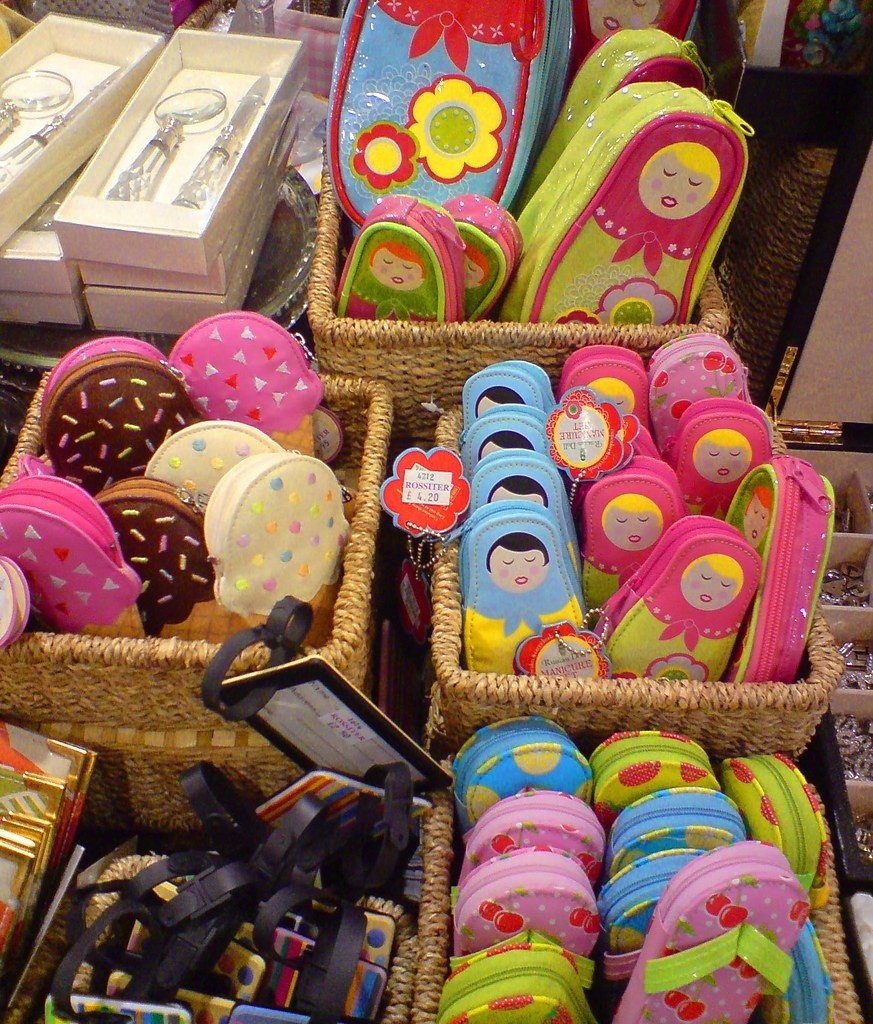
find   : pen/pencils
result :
[170,75,270,210]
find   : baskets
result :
[0,723,453,1024]
[308,118,730,468]
[0,371,397,735]
[412,788,864,1024]
[423,404,844,784]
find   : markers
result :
[0,65,129,193]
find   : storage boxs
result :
[0,13,302,333]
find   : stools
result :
[0,166,322,456]
